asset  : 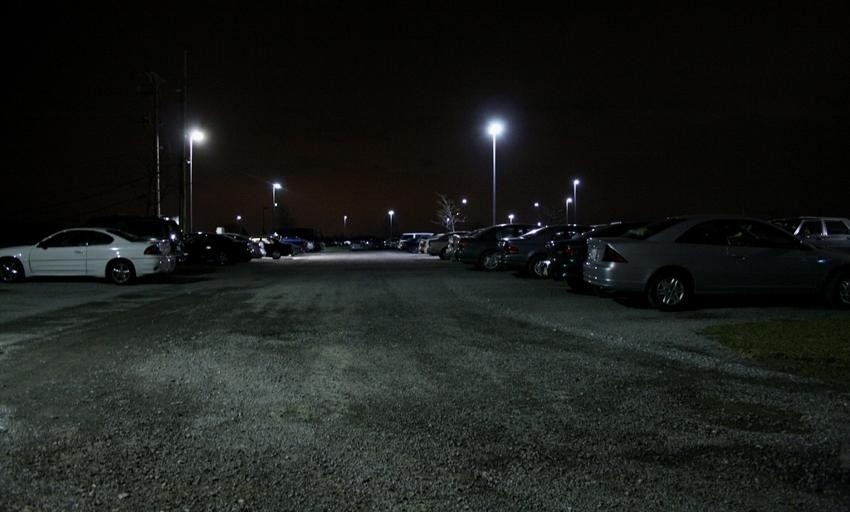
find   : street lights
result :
[237,216,242,235]
[343,216,347,241]
[463,120,580,225]
[189,129,206,231]
[273,182,281,232]
[388,210,394,244]
